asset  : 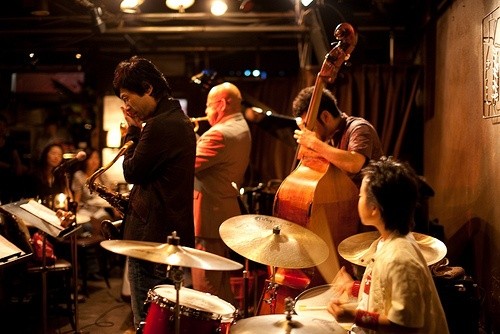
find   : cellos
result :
[255,22,357,315]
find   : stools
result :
[55,233,113,297]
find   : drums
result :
[139,284,237,334]
[292,284,359,330]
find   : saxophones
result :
[86,136,138,240]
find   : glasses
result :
[206,100,221,107]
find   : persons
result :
[194,81,251,304]
[327,156,449,334]
[34,144,100,302]
[293,86,383,189]
[113,55,196,334]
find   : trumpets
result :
[119,116,208,133]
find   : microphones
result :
[54,150,87,172]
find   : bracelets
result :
[352,280,360,297]
[355,308,380,330]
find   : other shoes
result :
[71,292,88,303]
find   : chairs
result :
[3,211,76,334]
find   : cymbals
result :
[100,239,244,270]
[230,313,348,334]
[219,214,329,269]
[338,231,447,267]
